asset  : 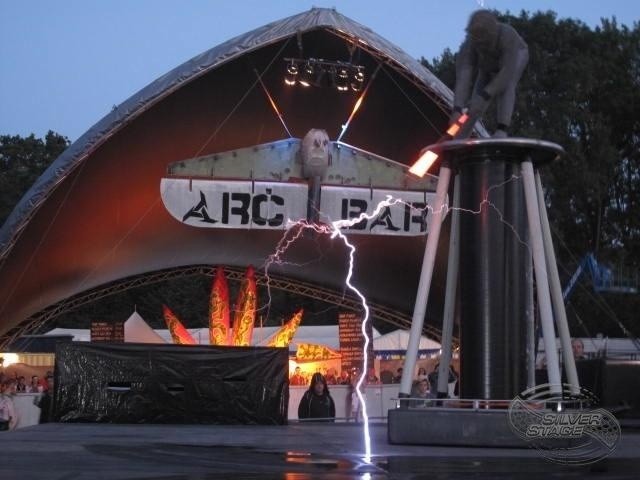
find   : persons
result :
[394,360,459,406]
[380,363,395,385]
[0,372,56,432]
[298,375,336,422]
[326,368,382,385]
[290,367,305,385]
[571,340,585,360]
[350,370,366,418]
[451,8,531,137]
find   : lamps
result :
[284,57,366,93]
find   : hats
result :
[416,375,428,383]
